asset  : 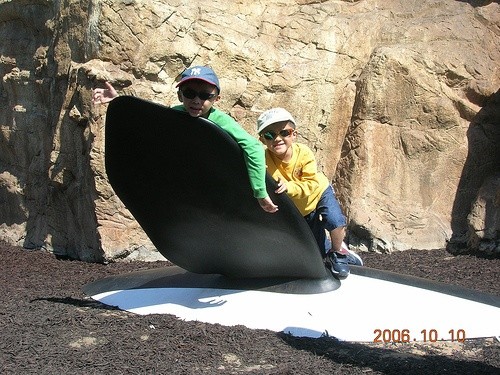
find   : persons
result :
[91,65,279,215]
[255,106,364,279]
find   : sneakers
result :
[340,242,364,266]
[327,248,350,277]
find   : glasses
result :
[178,90,219,100]
[260,129,296,141]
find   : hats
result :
[257,108,297,134]
[175,65,220,91]
